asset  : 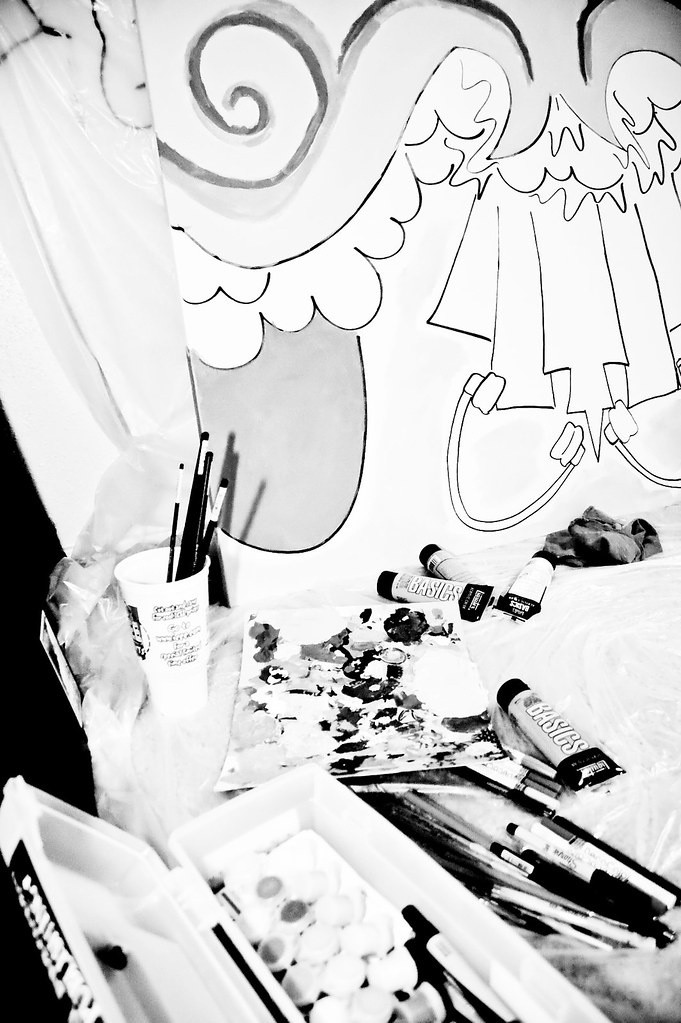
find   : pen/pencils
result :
[167,429,229,581]
[447,741,678,958]
[397,901,522,1023]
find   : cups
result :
[112,546,213,721]
